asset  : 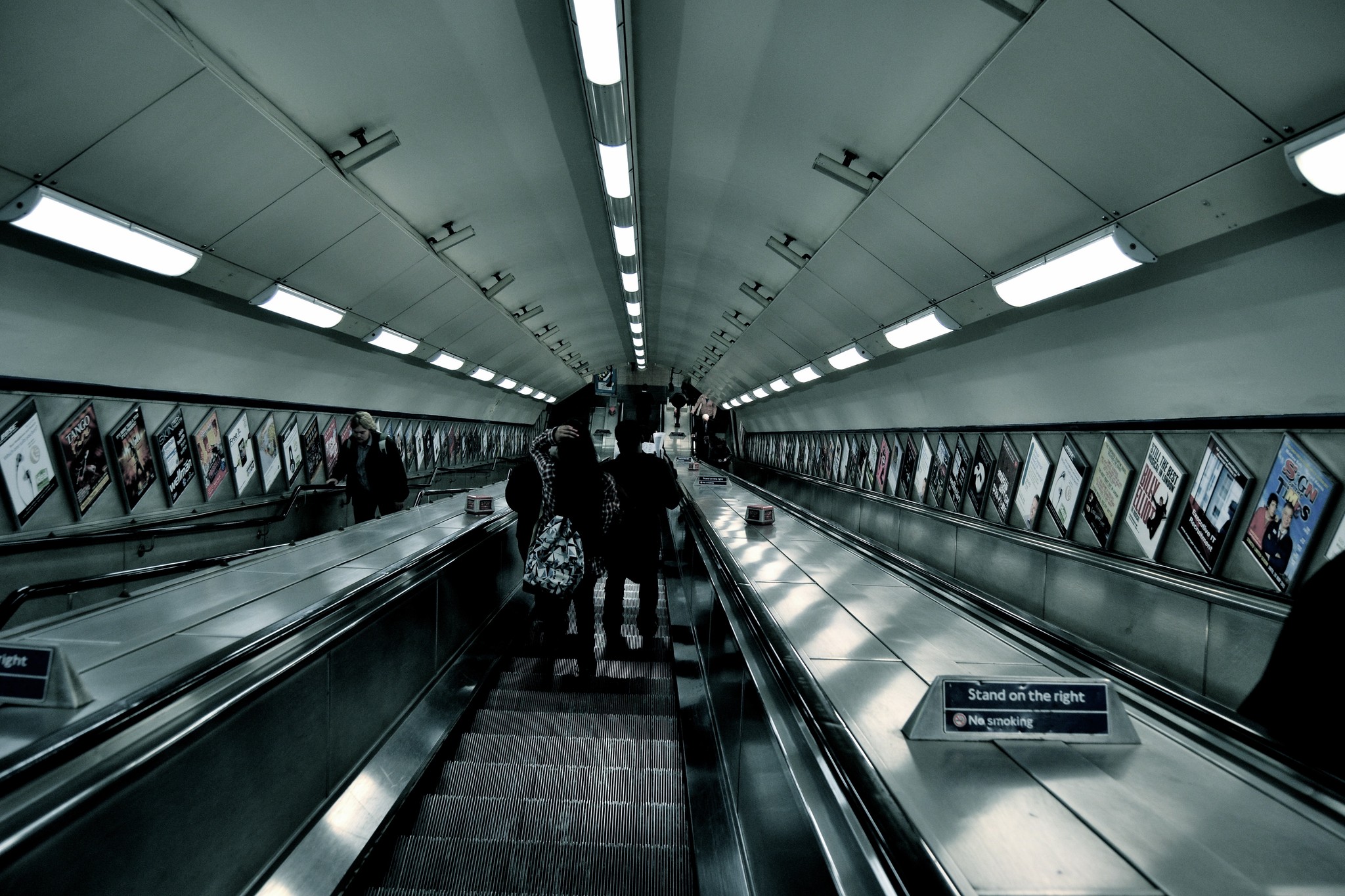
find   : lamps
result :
[1285,117,1345,201]
[769,375,794,392]
[0,182,204,279]
[753,385,773,398]
[543,395,557,403]
[424,350,466,371]
[883,305,963,349]
[825,342,875,370]
[514,383,534,395]
[247,283,347,328]
[362,325,420,354]
[792,363,826,383]
[721,391,756,410]
[991,224,1161,308]
[564,0,651,373]
[493,376,518,389]
[530,390,547,399]
[465,365,496,381]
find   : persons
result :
[596,417,686,658]
[527,417,621,690]
[1146,489,1169,540]
[1023,494,1040,529]
[710,437,733,473]
[689,393,717,419]
[324,410,410,526]
[1262,501,1295,575]
[396,433,405,457]
[1248,493,1280,548]
[197,442,211,488]
[1234,549,1345,796]
[679,376,693,397]
[918,477,928,502]
[203,436,216,464]
[691,413,718,462]
[211,417,224,455]
[504,410,582,645]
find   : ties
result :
[703,422,706,432]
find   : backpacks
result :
[522,511,585,599]
